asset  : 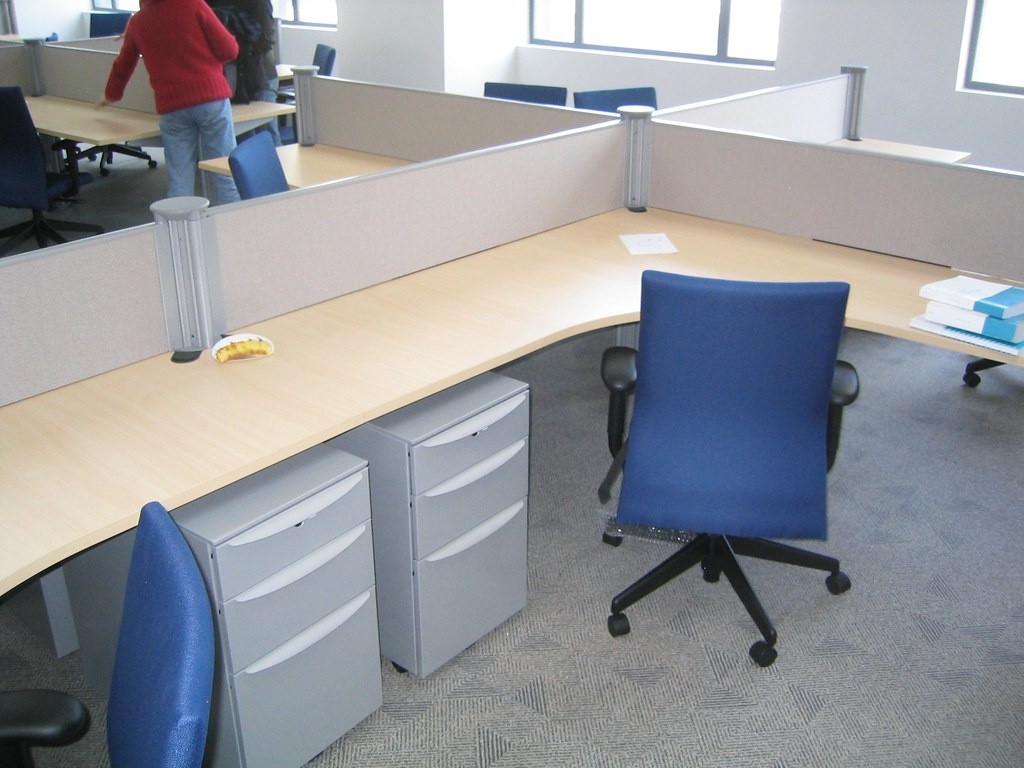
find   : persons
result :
[203,0,282,147]
[98,0,241,207]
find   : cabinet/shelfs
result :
[327,372,531,679]
[62,447,384,768]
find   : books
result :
[909,275,1024,357]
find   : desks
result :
[0,37,1024,605]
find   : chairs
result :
[64,144,157,174]
[600,268,860,668]
[0,501,216,768]
[227,131,291,200]
[277,44,336,127]
[574,87,658,113]
[0,85,104,264]
[277,92,297,146]
[89,12,135,40]
[479,83,568,109]
